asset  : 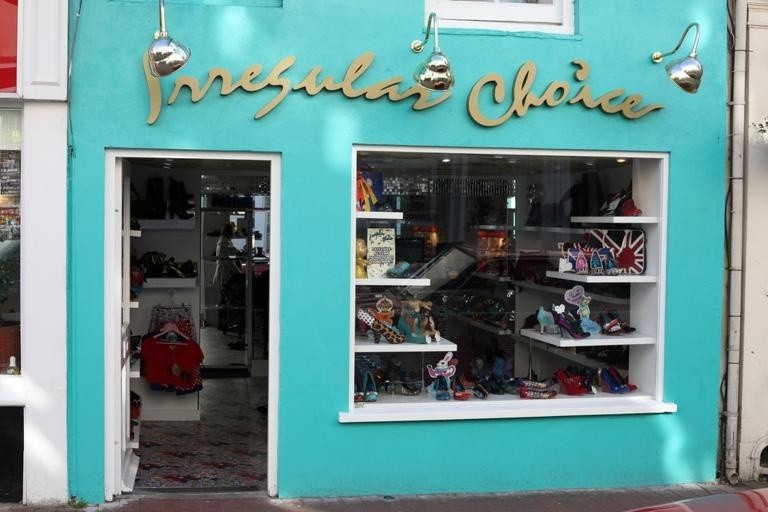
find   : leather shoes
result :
[618,199,641,216]
[436,375,450,399]
[452,376,470,399]
[519,378,557,398]
[575,250,619,275]
[206,229,262,238]
[168,261,196,278]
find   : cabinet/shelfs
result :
[120,179,203,491]
[353,206,658,398]
[203,179,250,372]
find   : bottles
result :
[7,355,20,375]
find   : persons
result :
[214,222,246,330]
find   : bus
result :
[371,220,439,259]
[469,224,594,284]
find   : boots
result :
[144,177,194,220]
[524,203,541,226]
[569,172,604,229]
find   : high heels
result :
[357,309,426,344]
[556,368,637,395]
[537,306,636,339]
[355,371,419,401]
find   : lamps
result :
[410,12,455,91]
[651,20,703,95]
[147,0,190,79]
